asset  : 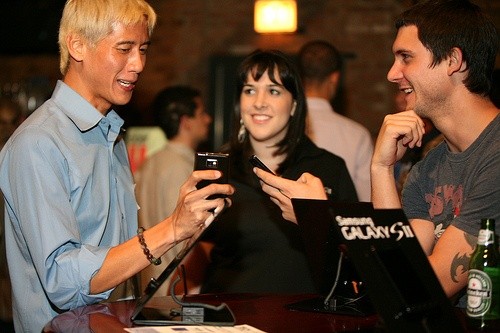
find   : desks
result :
[42,291,382,333]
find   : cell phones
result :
[249,154,277,176]
[193,152,230,213]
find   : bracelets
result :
[137,227,162,267]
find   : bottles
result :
[466,218,500,333]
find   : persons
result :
[194,48,362,300]
[367,4,499,310]
[131,84,213,300]
[369,89,444,207]
[298,40,376,204]
[0,0,236,333]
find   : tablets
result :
[292,198,463,333]
[130,197,231,320]
